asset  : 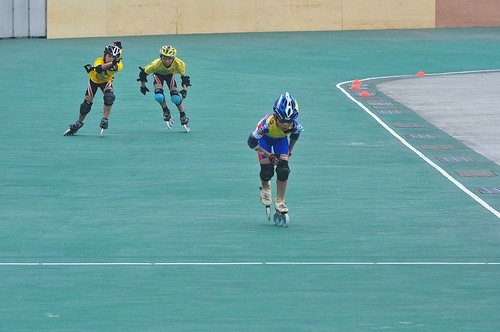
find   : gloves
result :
[112,57,121,65]
[140,86,150,95]
[180,90,187,99]
[269,154,281,166]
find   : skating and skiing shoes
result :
[63,120,84,136]
[180,115,191,133]
[273,198,290,228]
[162,109,174,129]
[99,118,108,136]
[260,180,272,221]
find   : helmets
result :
[273,92,299,121]
[104,45,123,60]
[159,45,177,59]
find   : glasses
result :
[276,116,294,123]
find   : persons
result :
[137,45,193,125]
[247,91,306,213]
[69,40,124,131]
